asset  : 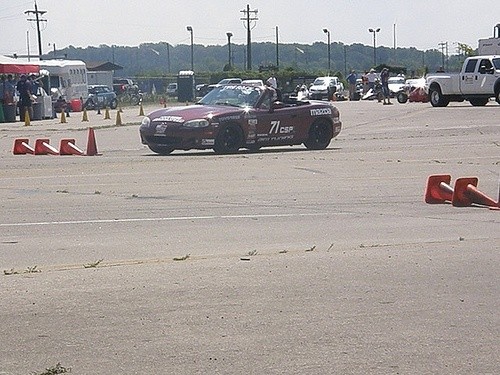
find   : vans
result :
[166,82,178,96]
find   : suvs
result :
[195,78,278,105]
[113,79,142,106]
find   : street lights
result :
[226,32,233,67]
[187,26,194,71]
[369,27,381,66]
[323,28,331,70]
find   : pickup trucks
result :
[426,55,500,107]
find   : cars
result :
[35,87,71,112]
[355,77,430,103]
[84,84,119,109]
[308,76,345,100]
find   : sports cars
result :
[138,84,342,154]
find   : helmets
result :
[259,87,277,104]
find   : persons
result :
[436,66,445,73]
[346,69,357,101]
[396,69,406,79]
[365,68,379,90]
[266,73,278,94]
[0,68,47,123]
[380,67,393,105]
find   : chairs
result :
[275,89,282,103]
[246,90,260,106]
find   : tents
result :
[0,52,41,85]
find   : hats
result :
[370,69,376,73]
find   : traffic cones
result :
[425,175,500,208]
[13,138,84,155]
[82,108,90,122]
[104,109,111,119]
[60,108,68,123]
[138,106,145,116]
[114,111,125,126]
[83,127,103,156]
[23,107,33,126]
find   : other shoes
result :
[383,102,393,105]
